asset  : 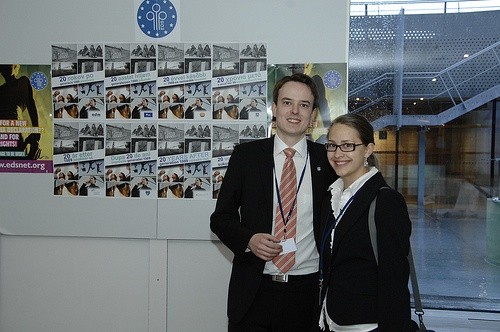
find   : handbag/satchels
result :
[416,321,435,332]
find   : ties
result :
[272,148,297,273]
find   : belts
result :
[262,271,319,283]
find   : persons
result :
[210,73,341,332]
[51,42,267,200]
[318,113,412,332]
[0,64,42,161]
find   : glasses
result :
[325,142,368,152]
